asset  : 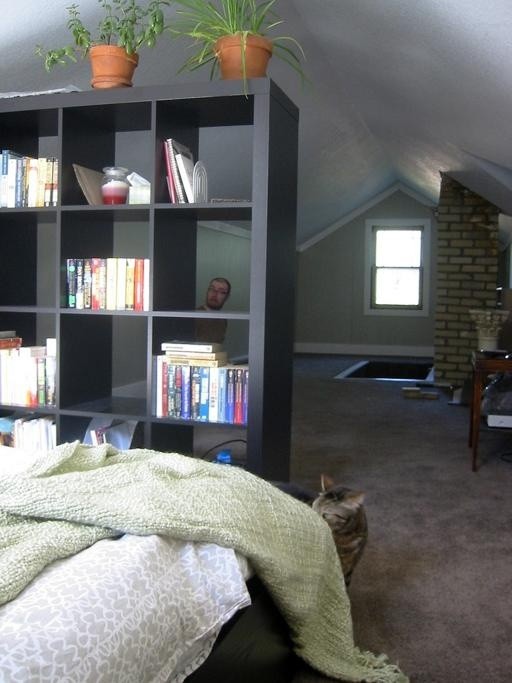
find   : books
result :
[1,139,206,311]
[0,329,254,453]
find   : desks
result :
[467,350,511,473]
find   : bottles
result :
[100,167,132,204]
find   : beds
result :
[1,441,345,682]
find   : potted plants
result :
[167,0,317,106]
[34,0,174,92]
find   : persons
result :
[191,279,236,345]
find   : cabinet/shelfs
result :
[1,76,307,495]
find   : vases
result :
[99,163,130,206]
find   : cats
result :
[265,475,369,587]
